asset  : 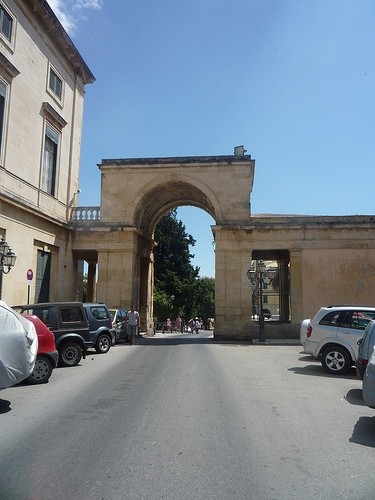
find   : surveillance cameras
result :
[77,189,81,193]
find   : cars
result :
[0,301,36,391]
[357,320,375,378]
[83,303,116,354]
[104,308,134,348]
[20,315,59,386]
[361,344,375,410]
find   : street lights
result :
[247,259,276,342]
[0,239,16,299]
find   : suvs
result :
[299,304,375,374]
[13,302,91,368]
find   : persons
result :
[125,305,141,345]
[165,315,202,334]
